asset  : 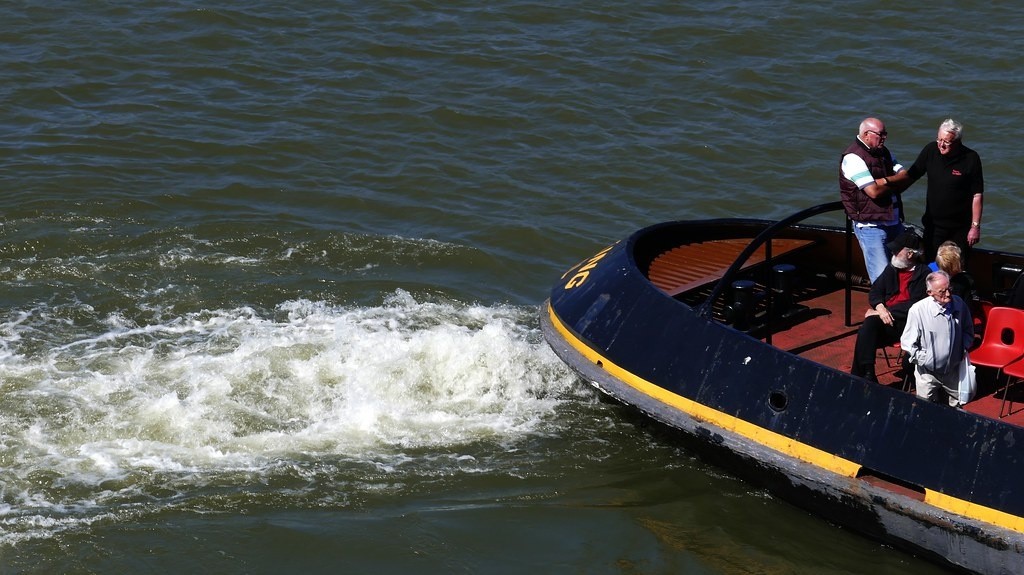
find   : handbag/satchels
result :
[958,349,990,406]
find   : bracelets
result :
[883,177,890,185]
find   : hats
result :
[886,233,920,252]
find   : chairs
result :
[880,300,1024,418]
[992,272,1024,305]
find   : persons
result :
[928,241,985,351]
[836,119,985,285]
[850,232,933,383]
[899,269,976,411]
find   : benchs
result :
[647,238,815,299]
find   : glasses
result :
[868,129,888,137]
[933,287,953,295]
[936,138,955,146]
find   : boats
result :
[540,196,1024,575]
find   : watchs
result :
[971,221,981,229]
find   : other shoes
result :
[864,371,878,384]
